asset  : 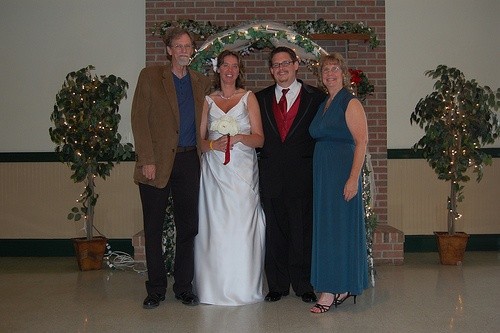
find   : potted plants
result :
[410,65,500,265]
[49,65,133,271]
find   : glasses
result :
[323,66,342,72]
[173,44,195,50]
[272,61,293,69]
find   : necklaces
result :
[218,88,237,99]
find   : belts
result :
[175,146,196,153]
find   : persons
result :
[255,47,329,302]
[131,29,212,308]
[192,49,270,306]
[309,52,370,313]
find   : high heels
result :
[310,292,340,313]
[332,291,358,305]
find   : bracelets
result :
[210,140,213,151]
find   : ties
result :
[280,89,290,115]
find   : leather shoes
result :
[264,291,289,301]
[295,292,318,303]
[176,291,200,305]
[143,293,166,307]
